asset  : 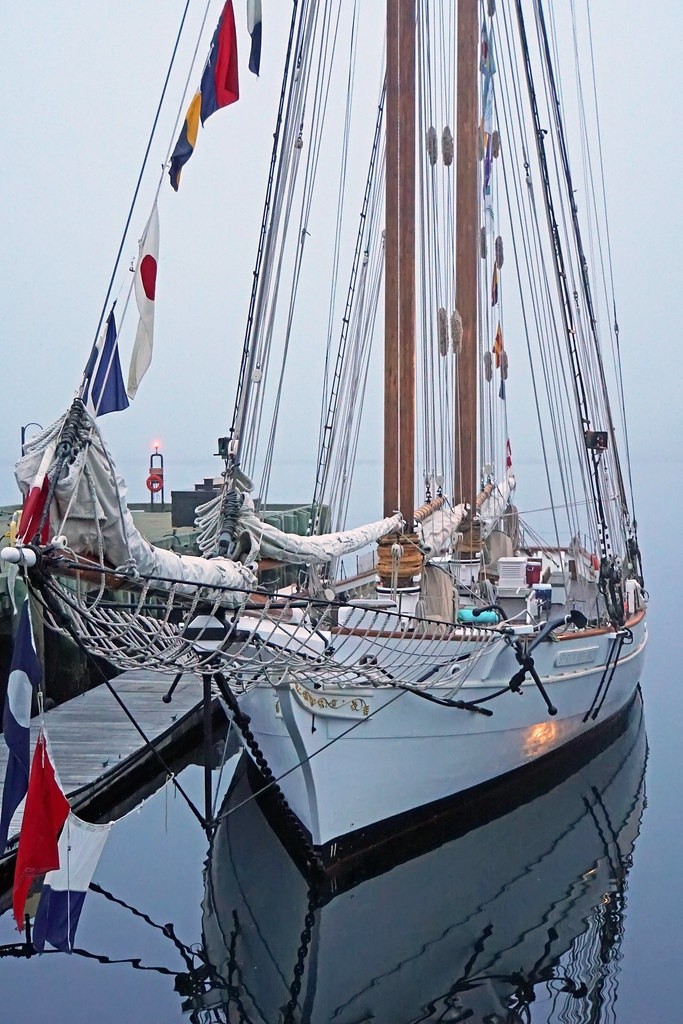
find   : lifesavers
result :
[146,474,162,492]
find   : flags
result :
[79,0,262,415]
[0,561,109,954]
[477,0,511,473]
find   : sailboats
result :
[157,679,650,1024]
[2,0,649,856]
